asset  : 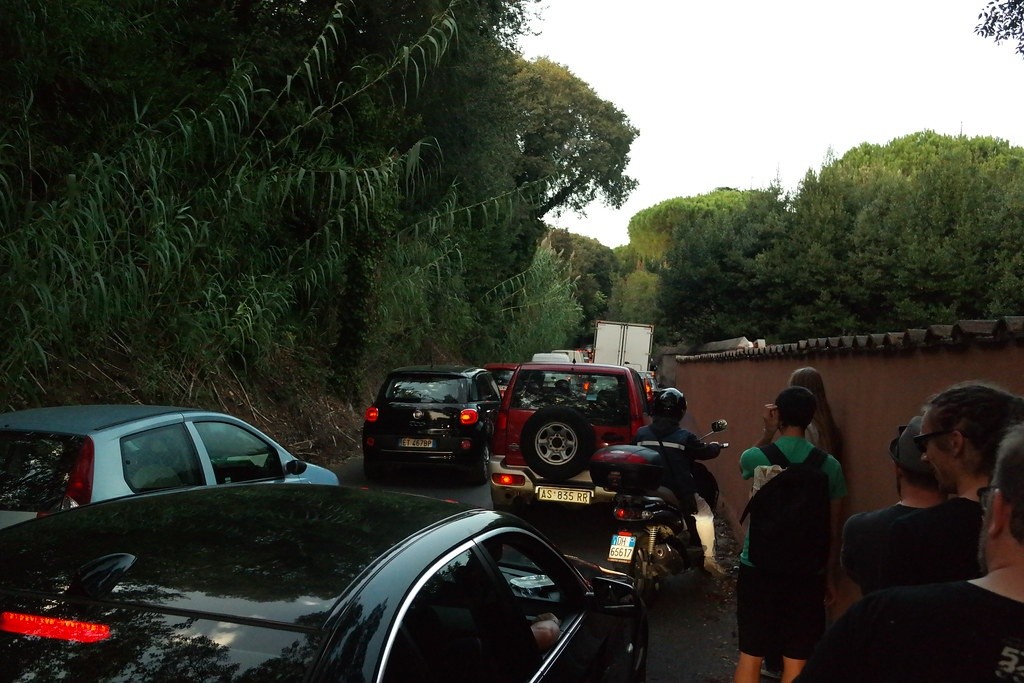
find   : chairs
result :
[130,463,185,491]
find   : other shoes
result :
[757,660,782,679]
[704,555,731,582]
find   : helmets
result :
[651,388,688,426]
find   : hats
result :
[885,416,942,481]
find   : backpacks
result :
[740,440,835,570]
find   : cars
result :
[483,348,662,406]
[0,483,652,683]
[0,404,339,529]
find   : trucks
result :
[589,318,655,391]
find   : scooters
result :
[587,418,731,609]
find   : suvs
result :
[481,359,654,519]
[357,363,502,484]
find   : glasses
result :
[976,484,998,511]
[913,431,947,454]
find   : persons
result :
[788,380,1024,683]
[630,387,728,581]
[531,613,559,649]
[734,387,846,683]
[788,367,844,461]
